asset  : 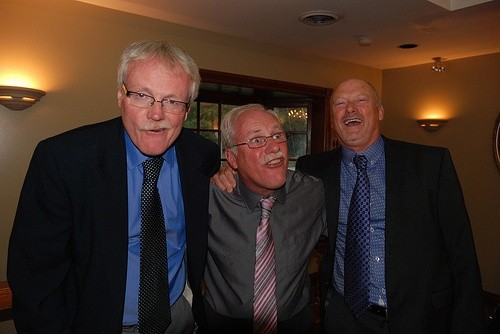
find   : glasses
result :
[122,82,191,114]
[223,131,287,149]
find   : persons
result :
[201,103,327,334]
[6,43,220,334]
[211,78,483,334]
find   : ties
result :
[252,197,278,334]
[342,155,371,317]
[138,156,172,334]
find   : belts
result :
[333,289,388,318]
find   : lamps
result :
[416,120,446,131]
[0,86,46,111]
